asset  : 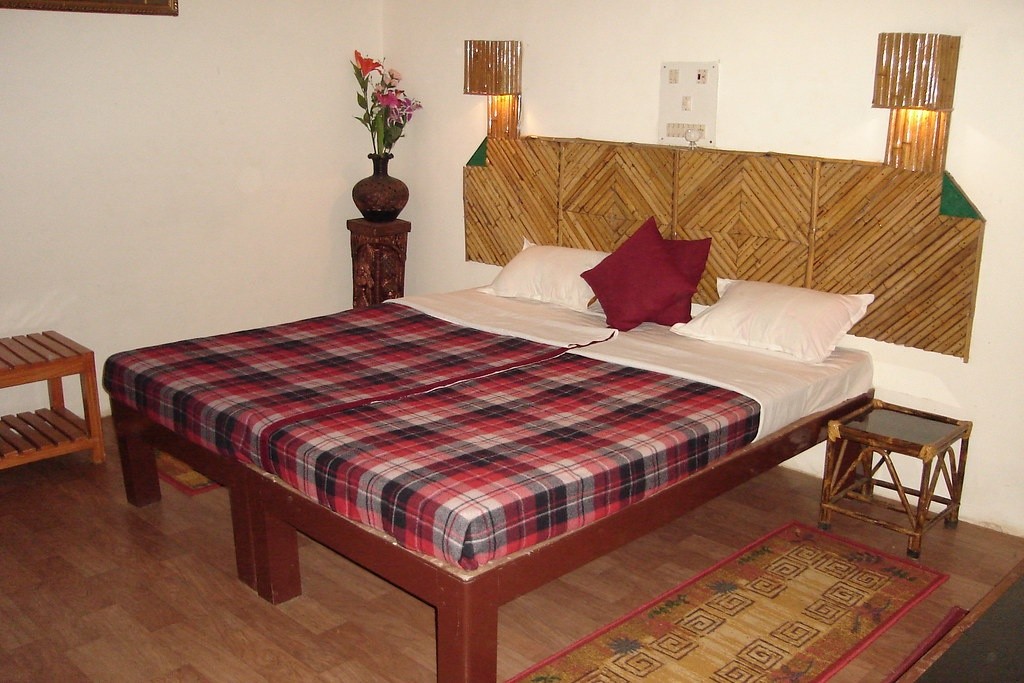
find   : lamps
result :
[464,41,522,140]
[872,32,960,172]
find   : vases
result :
[351,154,409,223]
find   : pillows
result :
[580,216,698,332]
[646,239,712,327]
[669,277,875,365]
[477,236,614,319]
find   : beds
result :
[102,136,986,683]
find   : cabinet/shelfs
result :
[0,331,106,470]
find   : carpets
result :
[503,519,951,683]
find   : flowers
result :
[350,49,422,155]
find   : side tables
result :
[817,398,973,560]
